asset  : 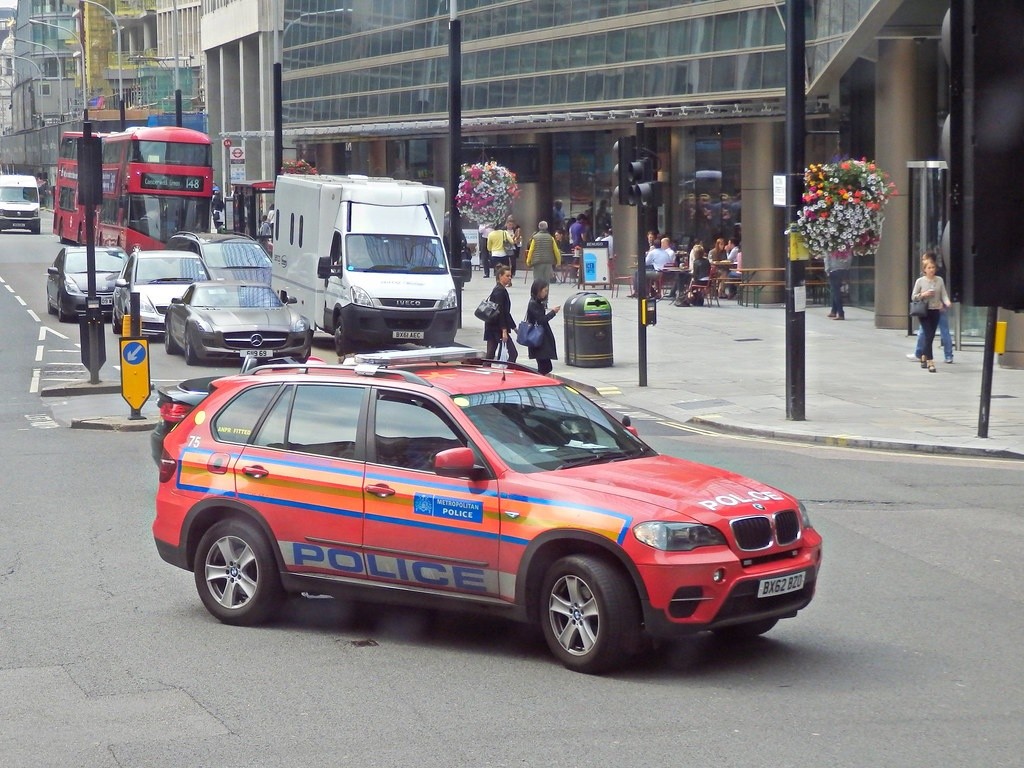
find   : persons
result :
[500,216,521,278]
[553,199,611,236]
[823,253,852,320]
[487,222,514,287]
[483,263,518,364]
[554,214,614,259]
[627,232,743,306]
[526,220,561,309]
[525,280,560,376]
[911,259,952,372]
[142,207,159,221]
[479,223,496,277]
[212,183,274,245]
[908,252,953,364]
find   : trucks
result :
[271,175,458,356]
[0,174,41,234]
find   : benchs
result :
[725,281,830,307]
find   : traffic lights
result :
[626,162,653,206]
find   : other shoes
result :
[627,291,676,300]
[906,354,921,361]
[921,355,935,372]
[943,358,953,362]
[827,313,844,320]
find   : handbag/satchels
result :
[503,230,516,256]
[499,340,509,368]
[517,301,544,349]
[910,294,927,317]
[674,291,704,307]
[475,290,503,324]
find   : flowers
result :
[791,159,890,256]
[454,159,521,222]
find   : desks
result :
[631,254,688,296]
[730,268,830,305]
[556,253,583,283]
[707,258,739,307]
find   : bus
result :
[53,131,119,246]
[95,128,214,256]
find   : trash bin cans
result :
[564,292,614,368]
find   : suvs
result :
[164,230,272,282]
[153,346,822,674]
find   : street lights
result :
[29,20,90,120]
[0,50,43,127]
[11,37,63,123]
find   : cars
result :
[164,280,312,366]
[46,246,130,322]
[112,250,214,340]
[151,354,599,468]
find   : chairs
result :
[557,256,804,308]
